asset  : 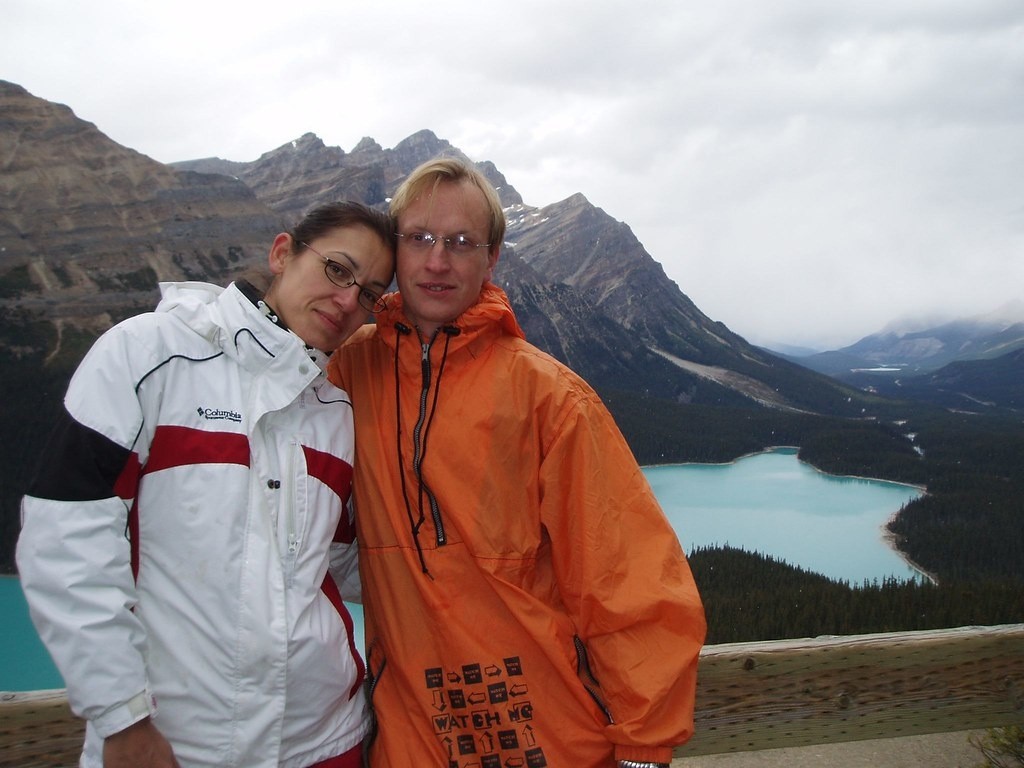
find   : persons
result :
[14,157,707,768]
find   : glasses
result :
[393,228,491,257]
[299,241,388,314]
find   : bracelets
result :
[619,758,656,767]
